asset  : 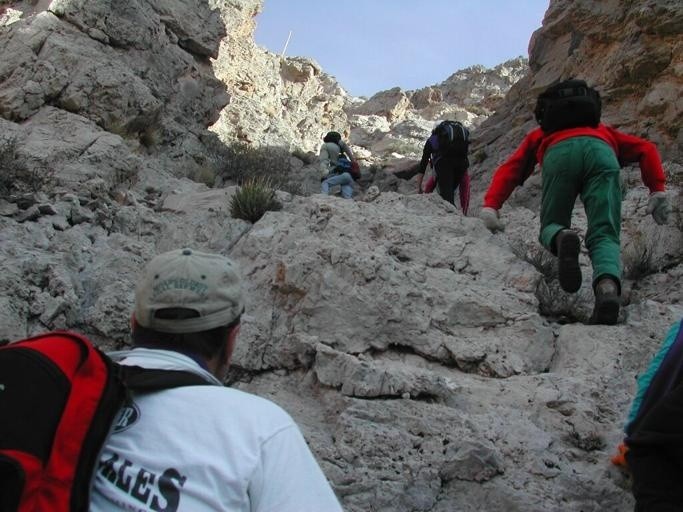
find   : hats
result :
[135,247,246,333]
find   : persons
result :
[608,317,682,511]
[318,130,359,201]
[480,120,671,326]
[86,246,343,512]
[414,118,470,218]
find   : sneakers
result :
[587,279,621,326]
[555,228,583,295]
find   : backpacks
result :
[350,161,362,182]
[532,78,603,136]
[431,119,470,145]
[323,131,341,145]
[0,330,214,512]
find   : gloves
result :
[644,190,673,226]
[479,207,505,235]
